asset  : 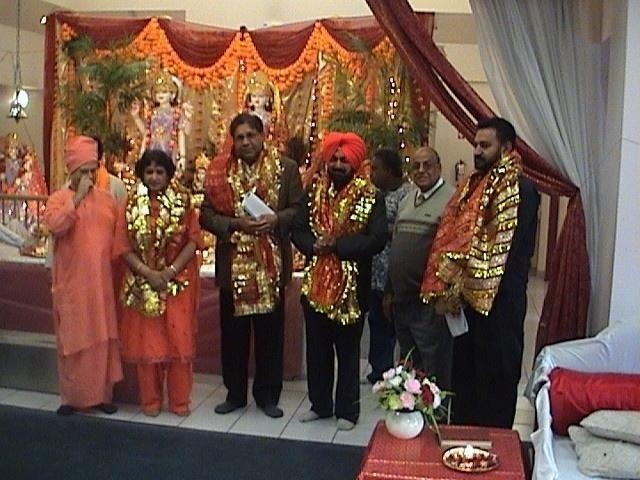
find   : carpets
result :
[2,403,366,480]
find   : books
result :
[437,426,495,450]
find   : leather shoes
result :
[214,400,237,414]
[257,404,283,418]
[336,417,355,430]
[92,403,118,414]
[298,410,320,422]
[57,405,73,415]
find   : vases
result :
[382,411,426,439]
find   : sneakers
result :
[172,401,190,418]
[144,401,161,418]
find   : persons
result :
[129,72,192,172]
[61,134,127,201]
[359,149,417,385]
[425,117,541,428]
[384,147,457,424]
[43,133,118,416]
[241,79,290,147]
[114,150,201,418]
[289,132,389,431]
[198,113,306,417]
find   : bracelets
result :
[164,264,178,280]
[132,261,144,275]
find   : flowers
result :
[351,339,458,451]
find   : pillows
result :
[581,410,640,446]
[545,368,640,435]
[568,424,640,479]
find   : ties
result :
[418,194,425,206]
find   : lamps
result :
[6,0,30,123]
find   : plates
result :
[441,446,501,472]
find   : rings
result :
[255,231,260,237]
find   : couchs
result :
[522,307,639,480]
